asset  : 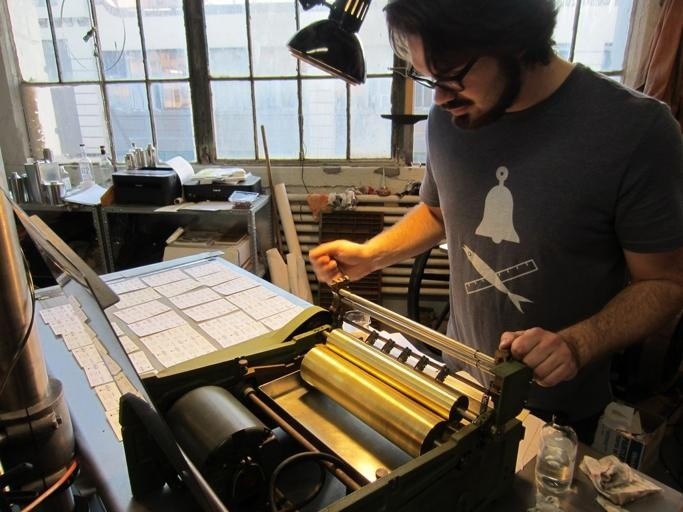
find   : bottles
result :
[36,146,72,191]
[76,143,97,185]
[122,142,158,169]
[6,154,67,204]
[98,144,116,186]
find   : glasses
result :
[406,42,488,93]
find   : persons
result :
[307,0,683,448]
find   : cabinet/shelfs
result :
[1,186,276,292]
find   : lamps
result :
[288,0,377,91]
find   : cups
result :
[534,422,580,496]
